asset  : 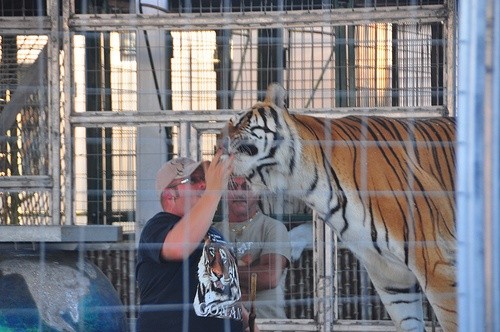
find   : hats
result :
[155,157,204,192]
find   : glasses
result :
[228,180,251,190]
[166,175,206,188]
